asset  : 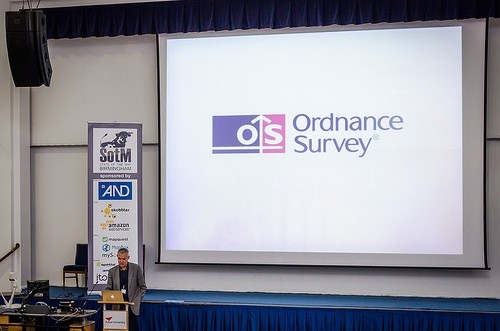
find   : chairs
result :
[63,244,88,290]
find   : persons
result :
[106,248,146,331]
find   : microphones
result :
[81,281,98,315]
[23,284,38,302]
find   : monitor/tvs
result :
[26,278,50,300]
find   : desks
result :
[139,301,500,331]
[0,307,98,331]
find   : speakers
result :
[5,10,52,88]
